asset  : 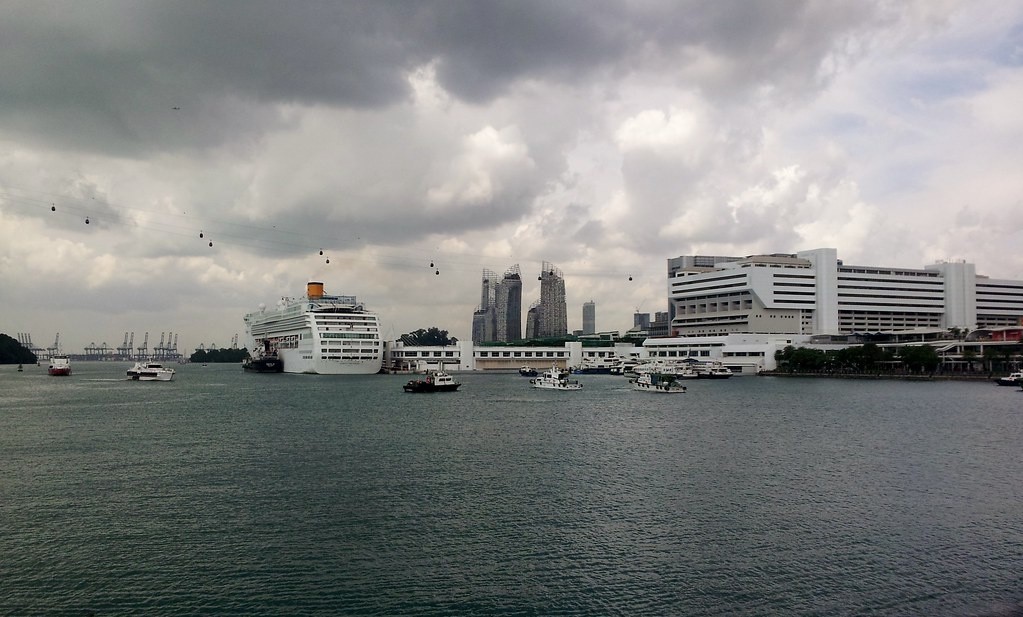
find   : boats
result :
[48,355,71,376]
[518,365,538,376]
[241,339,282,373]
[242,281,383,375]
[17,362,24,371]
[695,360,733,379]
[633,359,699,379]
[530,362,584,390]
[126,356,176,381]
[996,373,1023,386]
[403,371,462,393]
[628,372,687,393]
